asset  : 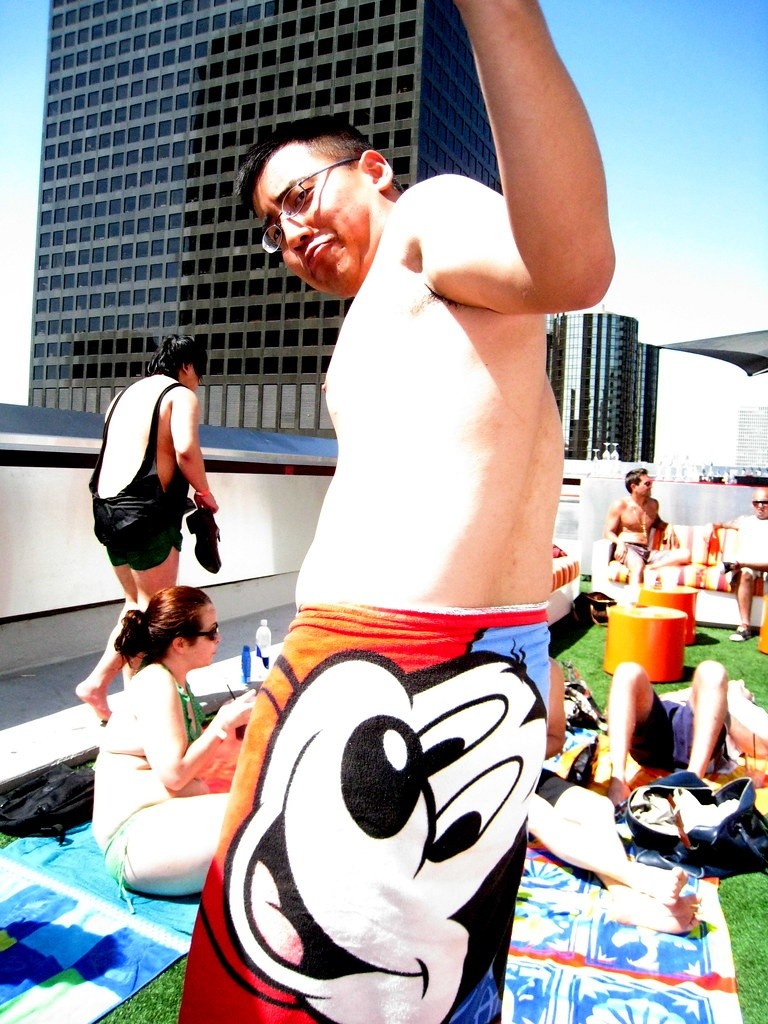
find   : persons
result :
[91,586,258,896]
[604,468,692,602]
[74,334,218,723]
[529,656,702,934]
[606,660,768,807]
[173,0,613,1024]
[720,487,768,642]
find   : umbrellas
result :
[653,329,768,377]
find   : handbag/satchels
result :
[626,770,768,870]
[571,592,617,626]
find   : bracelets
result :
[209,723,228,741]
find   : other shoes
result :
[185,507,223,575]
[729,625,753,641]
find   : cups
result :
[231,697,247,741]
[643,569,656,591]
[624,586,641,609]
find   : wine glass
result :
[593,443,619,460]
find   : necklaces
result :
[633,505,648,537]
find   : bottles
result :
[242,646,251,686]
[254,619,271,674]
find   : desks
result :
[579,476,768,581]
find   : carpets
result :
[0,727,743,1024]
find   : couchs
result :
[549,537,582,626]
[592,526,764,627]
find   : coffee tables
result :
[604,604,688,683]
[636,585,698,645]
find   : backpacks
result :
[0,762,94,837]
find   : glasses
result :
[751,500,768,506]
[262,156,360,253]
[174,623,221,640]
